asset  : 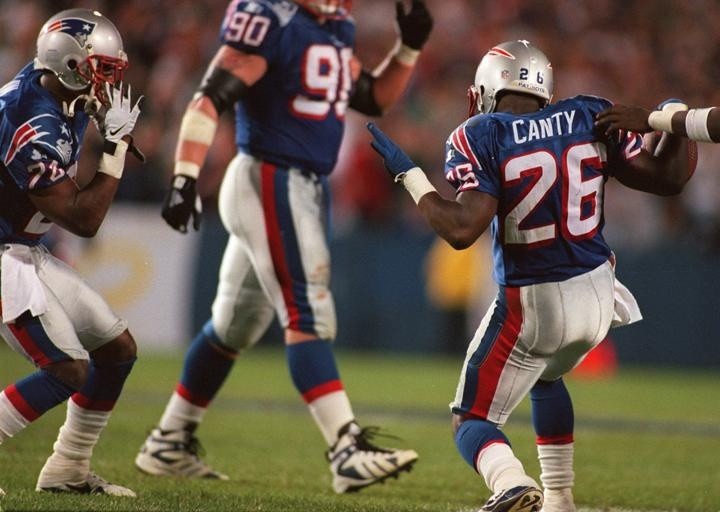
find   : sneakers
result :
[135,428,229,481]
[36,466,136,497]
[479,475,544,512]
[325,420,419,494]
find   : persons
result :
[133,0,434,495]
[0,7,147,497]
[594,103,720,144]
[366,42,698,511]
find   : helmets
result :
[468,40,553,118]
[34,9,124,90]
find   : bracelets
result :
[95,138,128,179]
[685,106,716,144]
[647,109,677,135]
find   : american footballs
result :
[643,128,698,197]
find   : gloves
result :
[367,121,418,184]
[657,99,689,111]
[161,175,202,233]
[84,81,145,179]
[396,0,433,51]
